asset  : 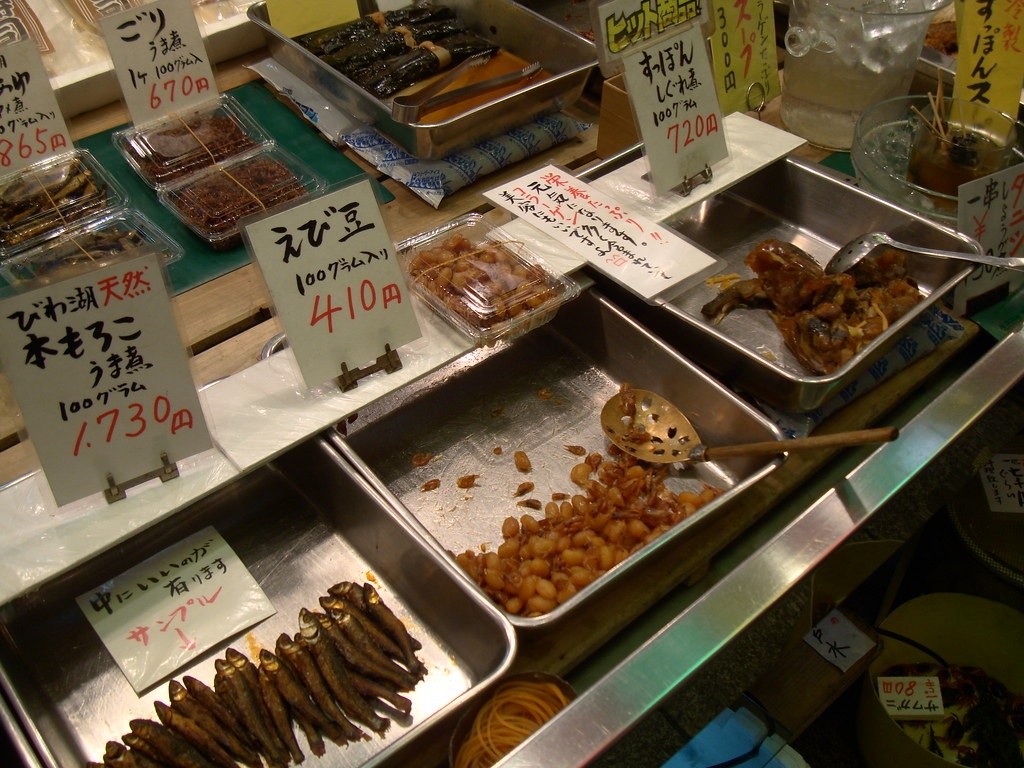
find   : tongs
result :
[391,47,543,124]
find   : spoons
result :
[823,231,1024,277]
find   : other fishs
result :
[76,577,425,768]
[0,160,161,301]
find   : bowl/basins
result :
[848,92,1024,232]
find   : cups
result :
[904,101,1017,198]
[780,1,952,152]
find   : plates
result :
[1,139,985,768]
[245,0,601,160]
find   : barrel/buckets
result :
[858,592,1023,767]
